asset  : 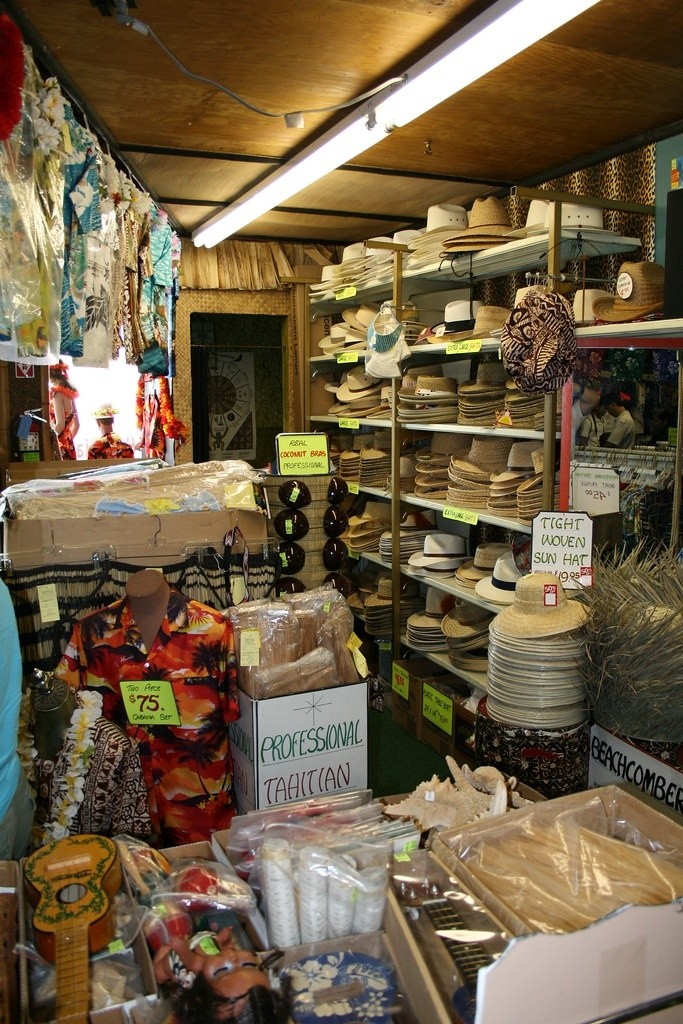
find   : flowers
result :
[14,686,101,848]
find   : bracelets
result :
[575,382,585,396]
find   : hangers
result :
[574,442,680,492]
[0,510,279,578]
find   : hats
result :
[594,259,667,324]
[316,262,578,396]
[321,364,575,435]
[486,574,596,736]
[344,494,533,605]
[346,571,489,673]
[319,426,560,524]
[305,198,629,306]
[93,404,119,419]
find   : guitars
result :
[21,834,123,1024]
[399,875,497,1024]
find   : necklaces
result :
[17,687,103,846]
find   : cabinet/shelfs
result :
[279,185,657,770]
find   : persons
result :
[579,404,608,447]
[48,359,80,460]
[16,672,152,860]
[0,576,36,862]
[53,568,243,852]
[86,404,134,459]
[599,392,636,449]
[152,925,292,1024]
[570,380,600,463]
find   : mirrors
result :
[558,338,683,572]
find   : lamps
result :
[189,1,602,249]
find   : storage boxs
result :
[3,456,270,569]
[0,768,682,1024]
[230,675,369,817]
[390,659,438,739]
[420,679,469,758]
[587,723,682,817]
[451,699,479,770]
